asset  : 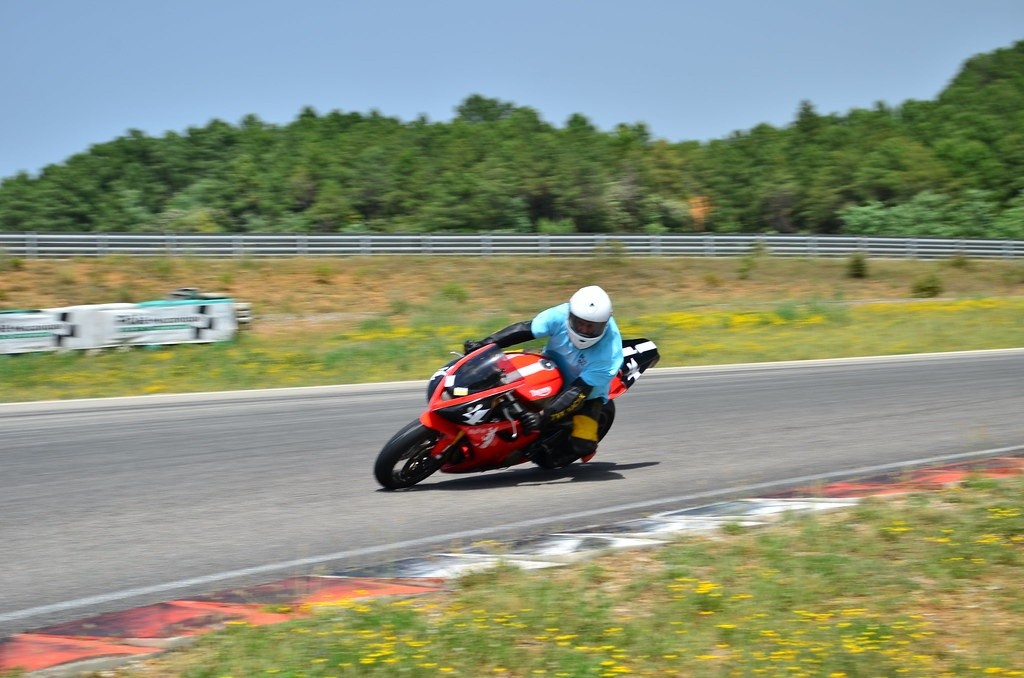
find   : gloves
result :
[520,411,542,433]
[464,340,484,355]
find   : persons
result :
[464,285,624,470]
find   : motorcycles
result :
[373,334,664,495]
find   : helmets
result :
[566,286,613,349]
